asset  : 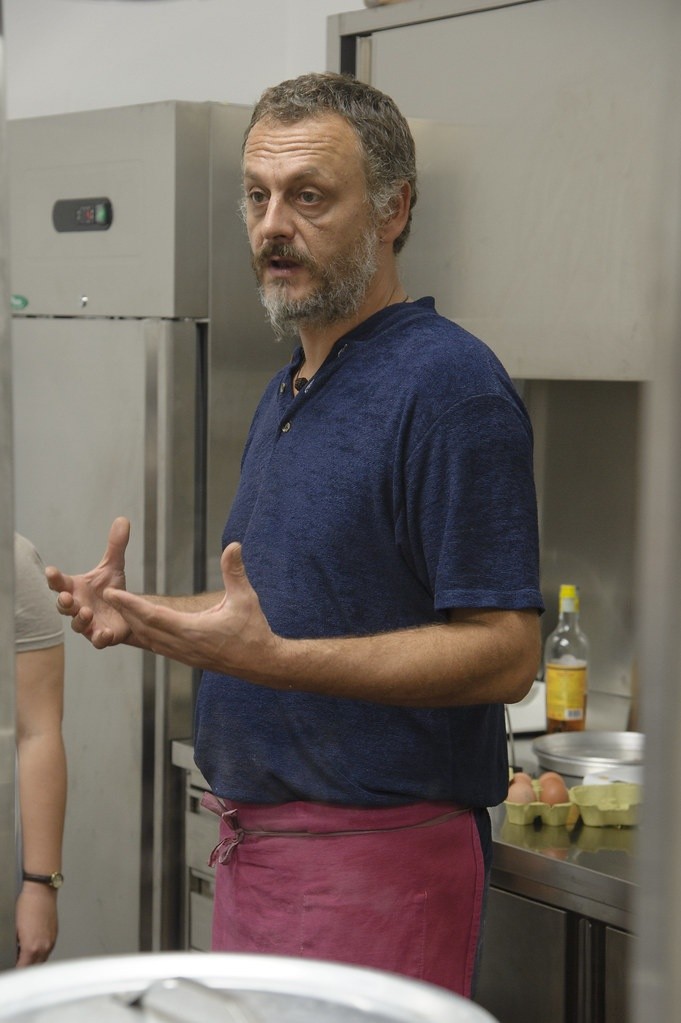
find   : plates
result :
[532,730,645,784]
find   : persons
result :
[41,74,545,997]
[10,530,66,968]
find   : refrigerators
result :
[2,102,303,972]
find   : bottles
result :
[544,585,590,733]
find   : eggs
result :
[505,771,570,804]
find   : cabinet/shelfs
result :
[183,770,633,1023]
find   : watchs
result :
[22,871,64,889]
